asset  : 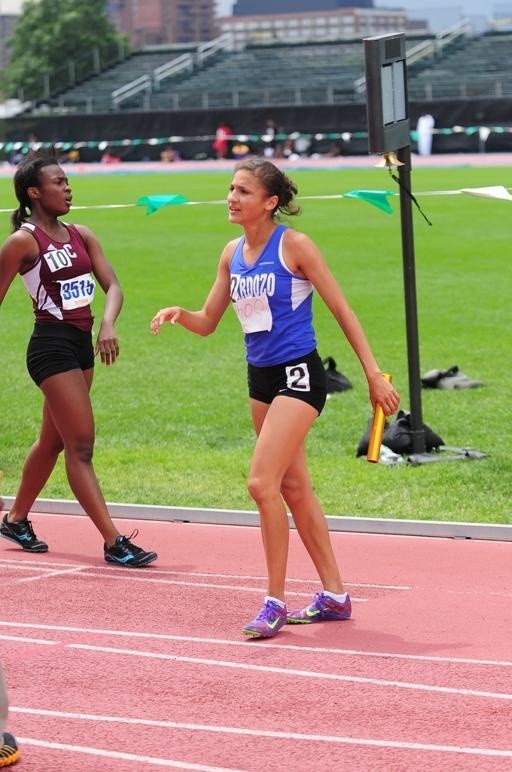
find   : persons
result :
[416,107,436,155]
[1,155,160,565]
[147,155,403,640]
[0,666,21,768]
[0,119,352,167]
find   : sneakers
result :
[1,733,19,767]
[103,529,159,567]
[0,514,49,552]
[241,600,287,637]
[287,592,352,624]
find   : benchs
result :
[20,28,512,118]
